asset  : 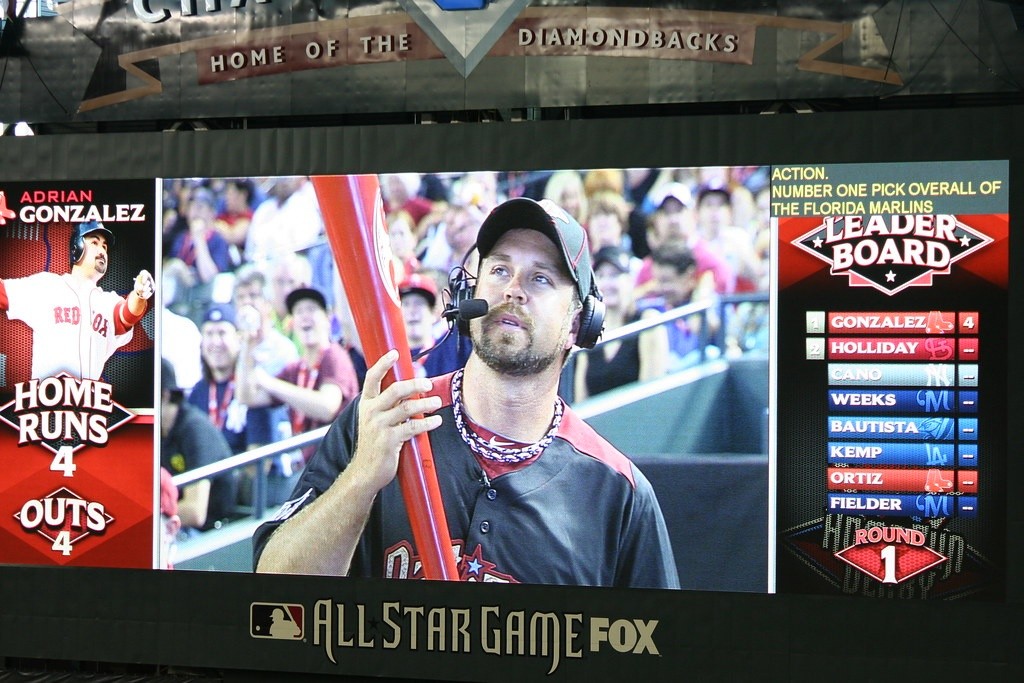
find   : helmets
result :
[70,220,115,263]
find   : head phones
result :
[450,243,606,350]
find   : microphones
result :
[442,300,488,320]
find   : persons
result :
[159,165,774,595]
[1,219,156,382]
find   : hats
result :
[592,246,629,272]
[286,288,325,313]
[698,179,729,206]
[477,196,591,303]
[398,273,438,308]
[655,182,692,210]
[202,306,237,332]
